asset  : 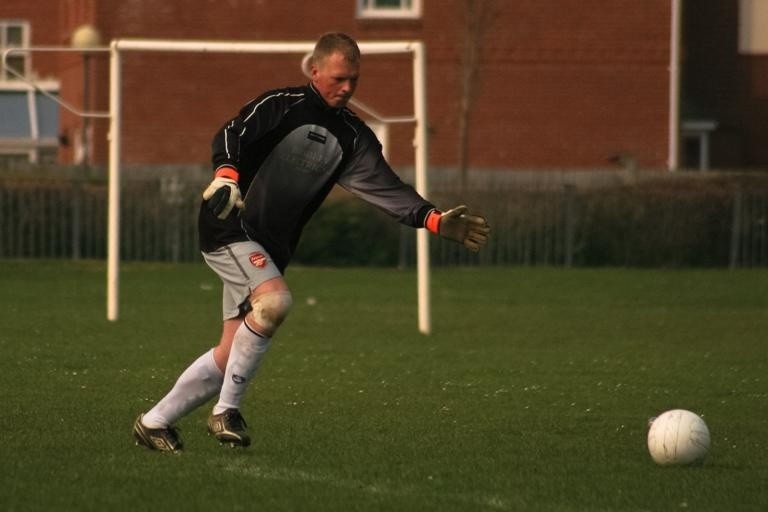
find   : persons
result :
[134,32,490,455]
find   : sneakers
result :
[208,408,251,448]
[133,413,184,455]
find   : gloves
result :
[423,204,489,253]
[203,177,245,220]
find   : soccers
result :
[648,410,710,466]
